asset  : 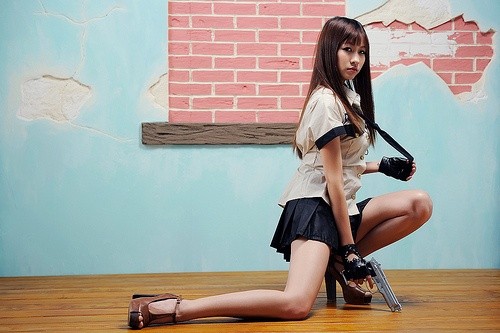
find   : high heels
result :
[325,259,372,304]
[128,293,181,329]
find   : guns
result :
[340,256,402,312]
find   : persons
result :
[127,16,434,330]
